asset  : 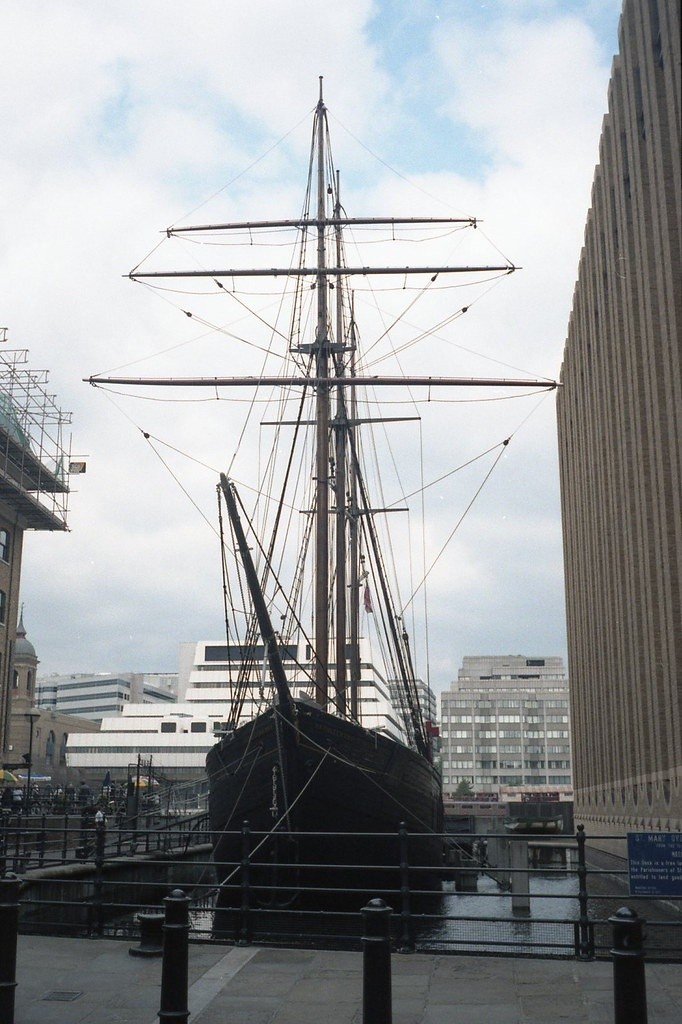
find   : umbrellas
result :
[123,775,159,786]
[103,770,112,797]
[0,769,18,782]
[18,772,51,781]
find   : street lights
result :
[21,708,42,865]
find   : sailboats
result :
[80,78,566,938]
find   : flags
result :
[364,582,373,613]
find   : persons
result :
[111,779,125,796]
[0,779,91,815]
[143,792,160,805]
[95,803,108,845]
